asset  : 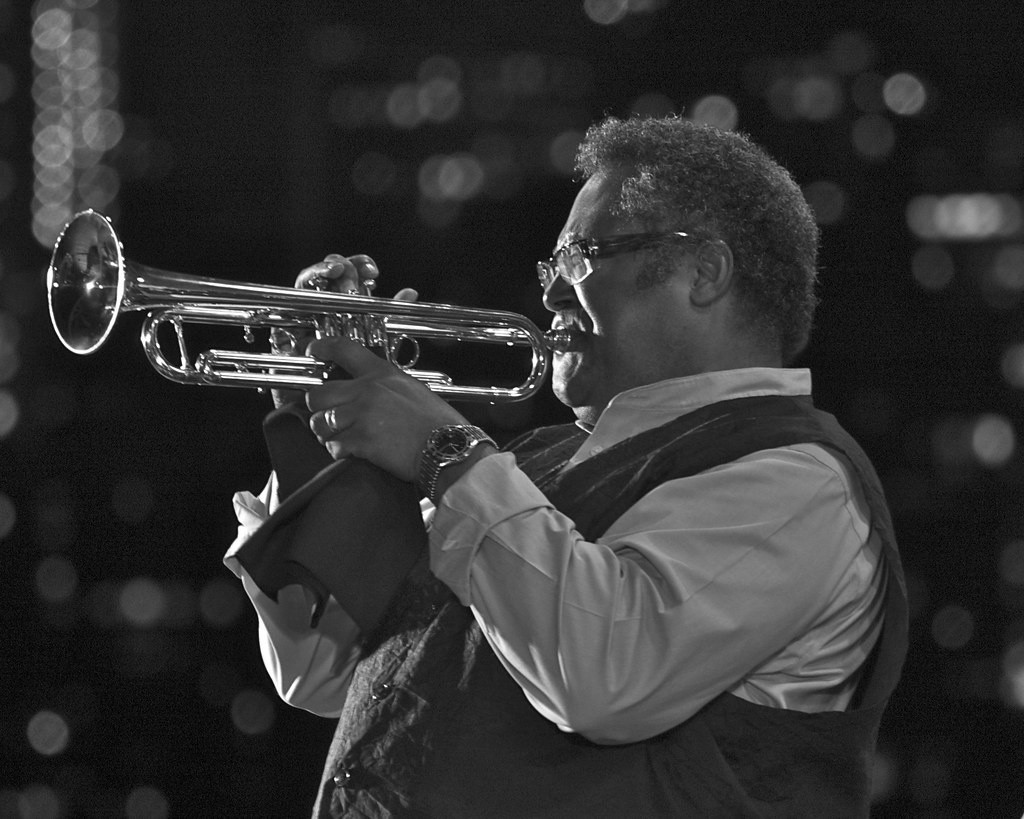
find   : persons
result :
[219,117,911,819]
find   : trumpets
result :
[43,211,576,405]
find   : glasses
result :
[536,230,711,294]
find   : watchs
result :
[416,424,500,501]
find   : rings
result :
[325,405,341,435]
